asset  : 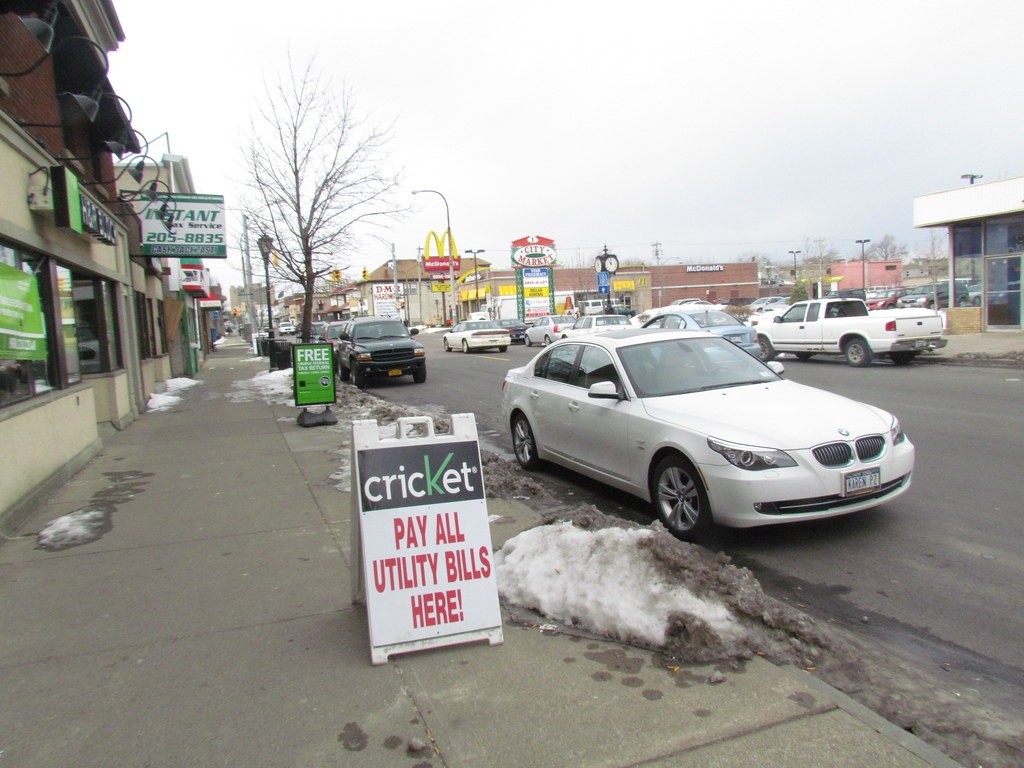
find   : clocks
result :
[595,257,602,272]
[604,257,618,272]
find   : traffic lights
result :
[362,269,368,281]
[333,270,341,281]
[232,307,238,317]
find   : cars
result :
[263,321,296,336]
[443,319,512,355]
[555,288,869,359]
[494,318,531,344]
[499,328,917,544]
[865,286,916,311]
[524,314,578,348]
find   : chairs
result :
[585,352,613,386]
[358,327,399,337]
[329,328,337,337]
[652,345,697,384]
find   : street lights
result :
[465,249,486,312]
[257,233,280,373]
[789,250,801,280]
[856,238,871,290]
[412,189,457,328]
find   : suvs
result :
[896,278,1020,312]
[298,319,352,370]
[336,316,427,390]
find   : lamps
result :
[0,36,110,122]
[56,127,148,184]
[84,155,160,203]
[115,194,177,230]
[102,180,171,221]
[15,0,60,53]
[18,92,132,161]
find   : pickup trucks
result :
[753,297,949,369]
